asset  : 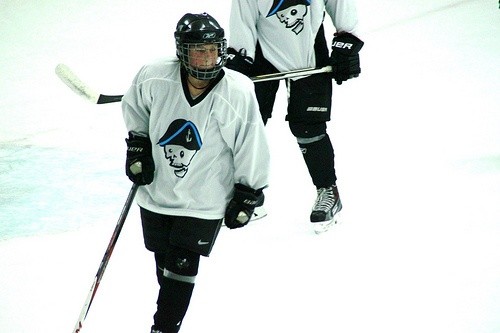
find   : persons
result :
[121,12,272,333]
[221,0,365,234]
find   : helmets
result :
[174,12,230,81]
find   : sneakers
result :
[308,181,343,234]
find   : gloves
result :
[124,137,178,190]
[224,48,254,78]
[329,31,364,86]
[224,184,270,229]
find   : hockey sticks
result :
[72,183,139,333]
[54,61,334,105]
